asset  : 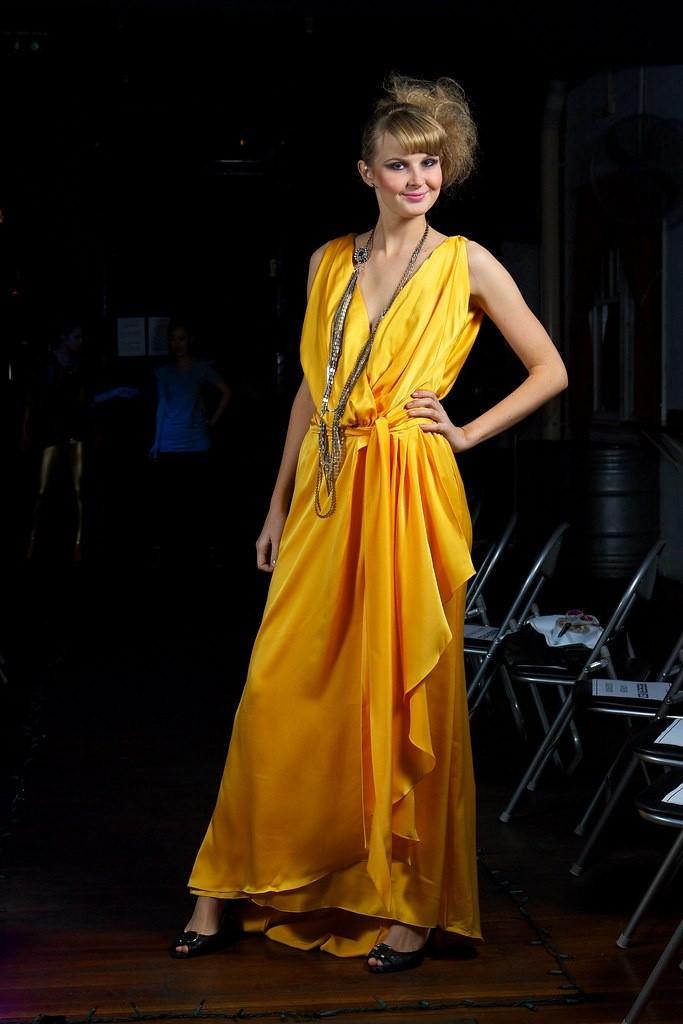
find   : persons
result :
[147,316,230,569]
[19,316,100,566]
[171,77,568,975]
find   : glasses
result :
[566,609,599,625]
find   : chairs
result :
[469,524,668,823]
[619,768,683,1024]
[568,635,683,878]
[614,716,683,948]
[463,503,527,742]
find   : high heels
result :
[366,923,435,972]
[171,898,242,958]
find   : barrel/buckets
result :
[577,450,655,586]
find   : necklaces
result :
[314,222,430,518]
